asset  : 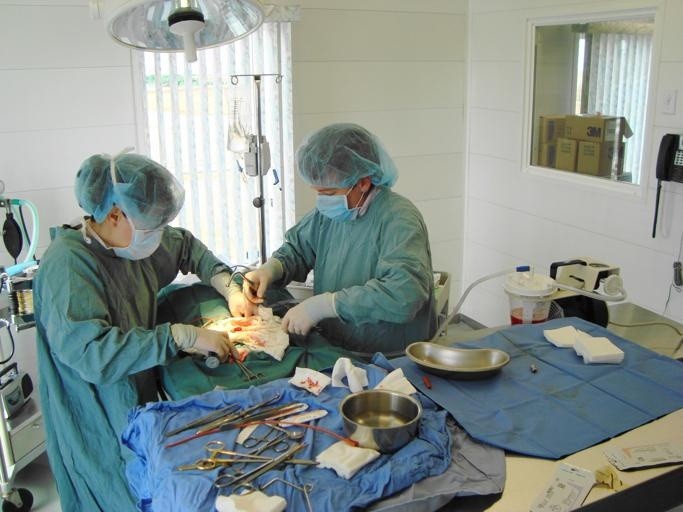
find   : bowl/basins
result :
[339,390,422,451]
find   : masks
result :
[110,228,165,263]
[314,191,363,224]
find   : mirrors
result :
[520,7,660,198]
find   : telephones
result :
[656,133,683,183]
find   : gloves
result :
[206,269,260,318]
[241,255,285,304]
[170,321,242,364]
[280,291,346,337]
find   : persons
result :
[232,121,433,359]
[27,145,259,512]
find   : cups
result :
[504,271,558,326]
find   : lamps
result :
[106,0,266,63]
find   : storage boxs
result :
[538,113,634,177]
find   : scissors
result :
[176,418,310,497]
[235,360,266,383]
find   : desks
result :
[143,317,683,512]
